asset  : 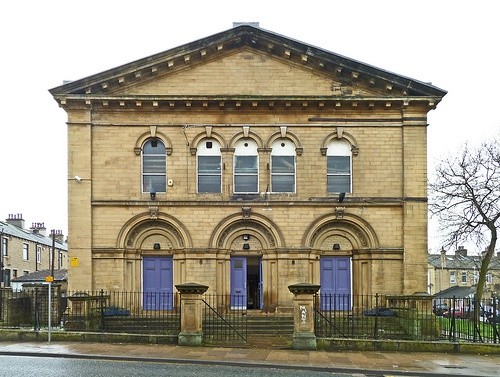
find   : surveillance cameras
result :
[75,176,82,183]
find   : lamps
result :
[339,192,345,201]
[154,243,160,249]
[332,243,340,250]
[243,234,250,240]
[243,243,249,249]
[150,191,156,200]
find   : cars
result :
[433,302,500,324]
